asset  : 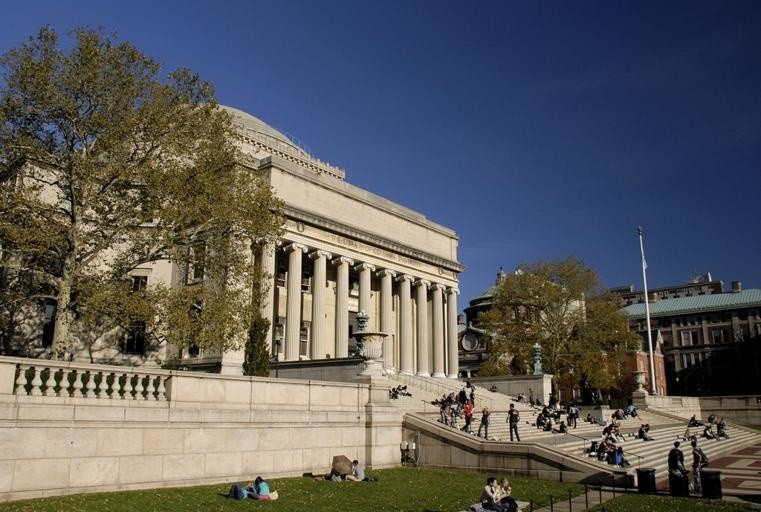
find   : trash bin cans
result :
[636,468,656,492]
[701,469,723,500]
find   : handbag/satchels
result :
[268,489,280,501]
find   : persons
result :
[691,441,708,493]
[345,459,365,481]
[668,441,694,491]
[506,404,520,442]
[431,385,491,440]
[499,476,519,512]
[587,402,655,468]
[519,387,579,433]
[684,414,730,442]
[490,385,497,392]
[481,477,508,511]
[391,384,412,400]
[229,482,257,500]
[248,475,270,500]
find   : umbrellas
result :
[333,454,353,474]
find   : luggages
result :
[694,449,709,467]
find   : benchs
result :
[472,500,530,511]
